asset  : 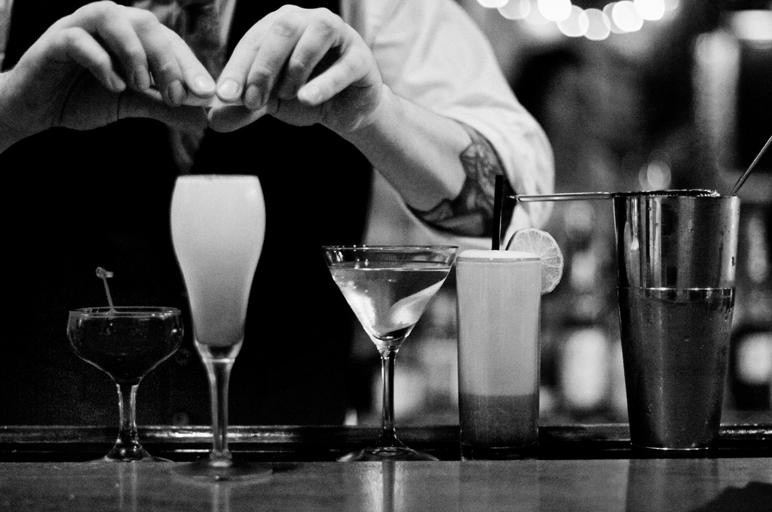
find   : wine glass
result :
[67,307,184,466]
[168,174,275,483]
[323,245,463,461]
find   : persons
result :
[2,0,558,445]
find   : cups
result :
[453,256,543,453]
[611,190,742,450]
